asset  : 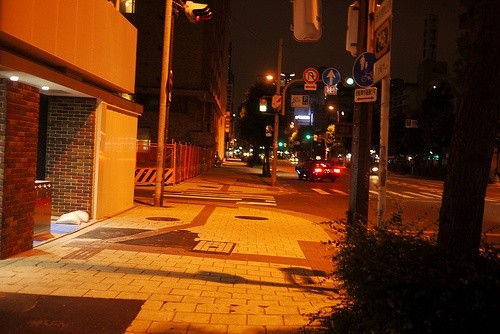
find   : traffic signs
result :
[352,51,378,103]
[372,0,391,84]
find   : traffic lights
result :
[279,142,283,147]
[344,76,356,88]
[306,135,311,140]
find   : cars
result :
[298,158,343,181]
[236,145,299,161]
[346,149,380,174]
